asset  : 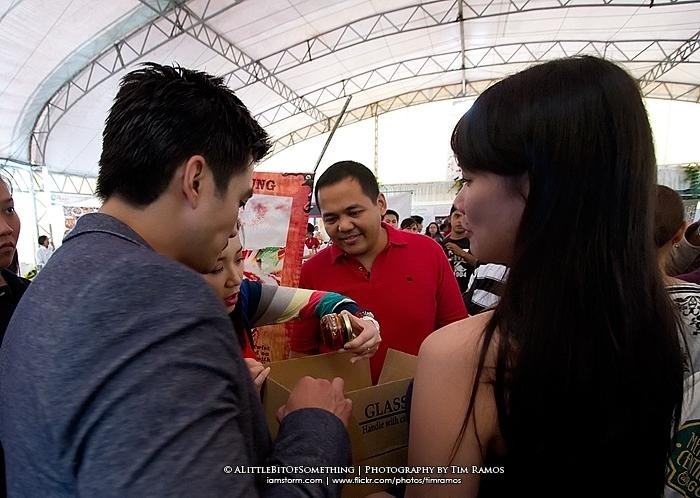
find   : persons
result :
[0,54,700,498]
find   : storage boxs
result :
[257,347,418,498]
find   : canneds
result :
[320,312,353,350]
